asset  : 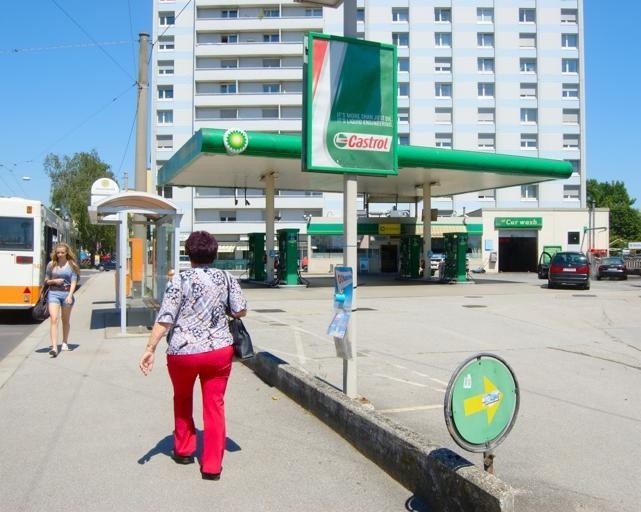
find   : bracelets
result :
[146,343,157,351]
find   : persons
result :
[138,229,250,480]
[41,242,80,356]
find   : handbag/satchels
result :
[32,285,51,322]
[228,317,255,361]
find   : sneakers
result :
[200,465,223,481]
[60,341,70,352]
[173,448,195,465]
[49,348,58,358]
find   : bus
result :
[0,197,70,324]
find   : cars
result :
[537,250,590,290]
[595,257,627,281]
[95,259,117,272]
[430,254,445,270]
